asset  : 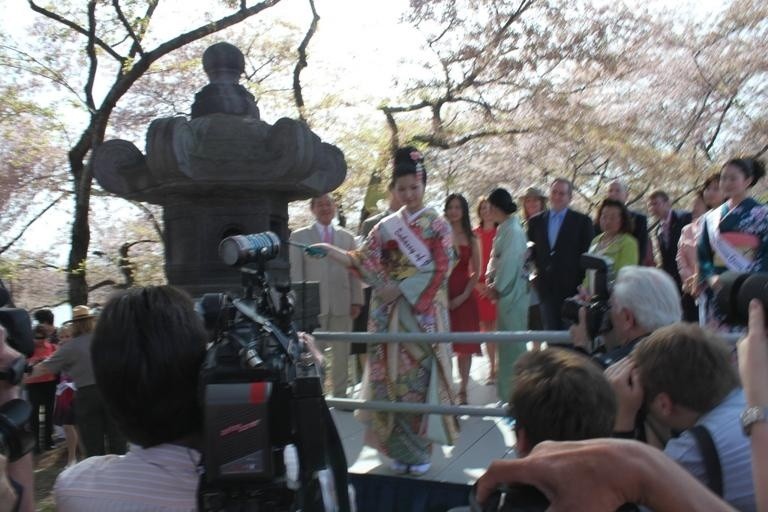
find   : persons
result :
[287,146,767,512]
[0,283,326,512]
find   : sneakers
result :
[391,461,431,477]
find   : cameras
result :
[718,271,768,326]
[0,399,37,463]
[560,252,613,329]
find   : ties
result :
[324,226,329,243]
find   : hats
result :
[63,305,97,327]
[518,188,548,200]
